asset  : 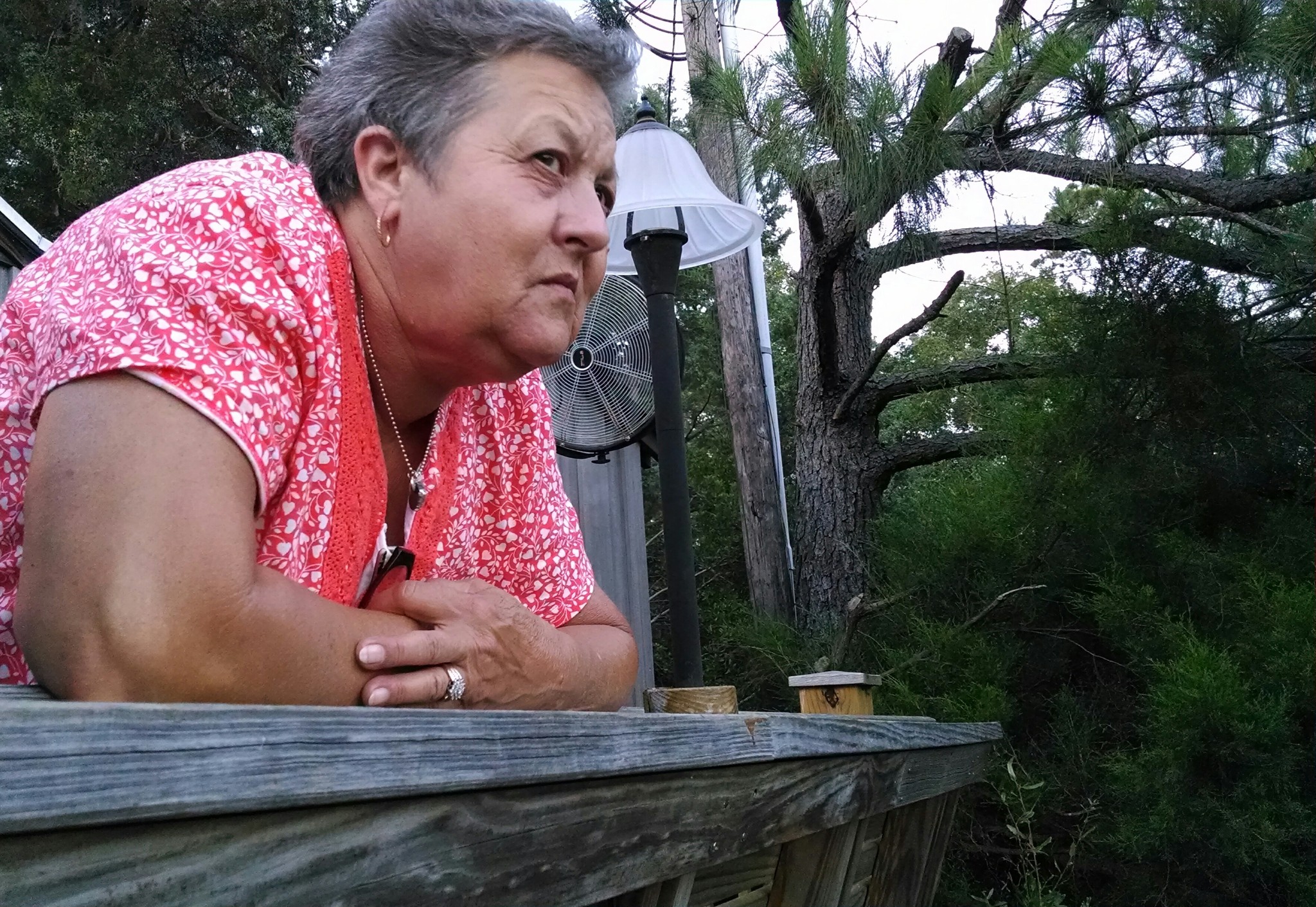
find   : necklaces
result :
[358,293,442,511]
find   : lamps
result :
[603,95,767,687]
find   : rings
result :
[440,664,467,702]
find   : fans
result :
[543,275,684,464]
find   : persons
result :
[0,0,639,709]
